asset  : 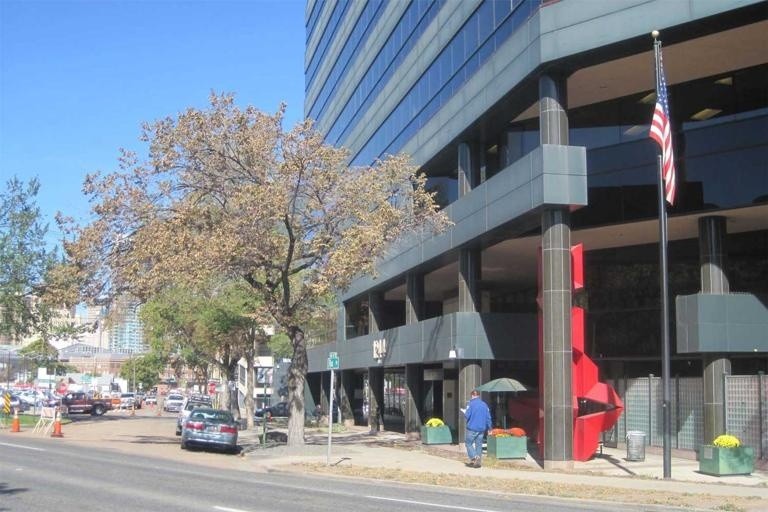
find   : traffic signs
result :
[327,352,340,370]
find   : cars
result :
[164,392,241,452]
[120,392,160,410]
[256,400,289,417]
[0,385,61,414]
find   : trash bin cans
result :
[626,431,647,461]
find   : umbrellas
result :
[472,377,527,392]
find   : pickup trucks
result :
[41,392,113,416]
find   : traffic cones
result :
[52,412,63,437]
[10,408,22,432]
[141,400,162,416]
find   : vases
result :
[698,443,754,476]
[419,425,452,444]
[486,434,527,459]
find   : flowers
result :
[487,427,527,438]
[712,434,741,447]
[426,417,445,426]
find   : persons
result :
[312,404,321,419]
[464,390,493,468]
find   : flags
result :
[649,41,677,207]
